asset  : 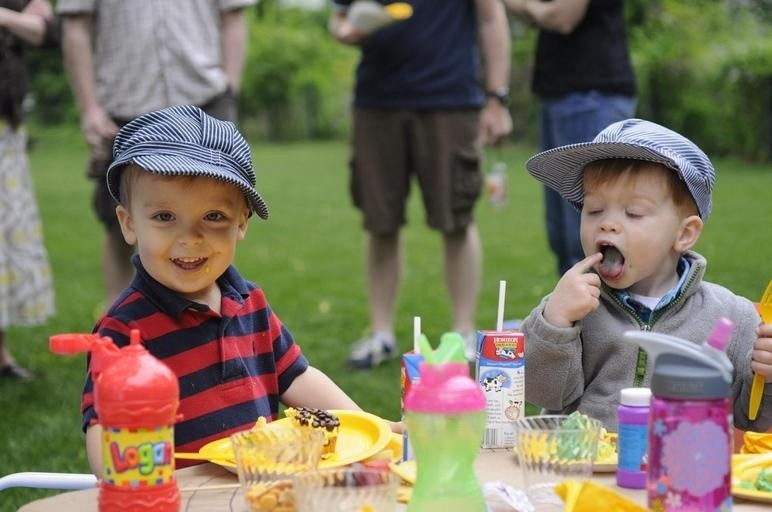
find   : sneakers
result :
[348,332,397,368]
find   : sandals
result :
[4,360,36,384]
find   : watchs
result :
[490,85,511,106]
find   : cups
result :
[292,467,400,511]
[230,427,325,512]
[514,414,603,491]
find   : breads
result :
[284,405,340,462]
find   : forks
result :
[748,280,772,420]
[173,451,239,464]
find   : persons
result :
[1,0,54,382]
[55,0,254,311]
[80,104,406,479]
[502,0,641,279]
[326,0,514,370]
[518,118,772,432]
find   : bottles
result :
[476,330,526,450]
[403,330,488,511]
[400,349,424,462]
[483,129,508,209]
[48,326,184,512]
[621,316,736,512]
[616,387,652,489]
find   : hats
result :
[525,118,717,219]
[107,103,270,220]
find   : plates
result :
[513,432,619,473]
[199,410,391,474]
[731,454,771,502]
